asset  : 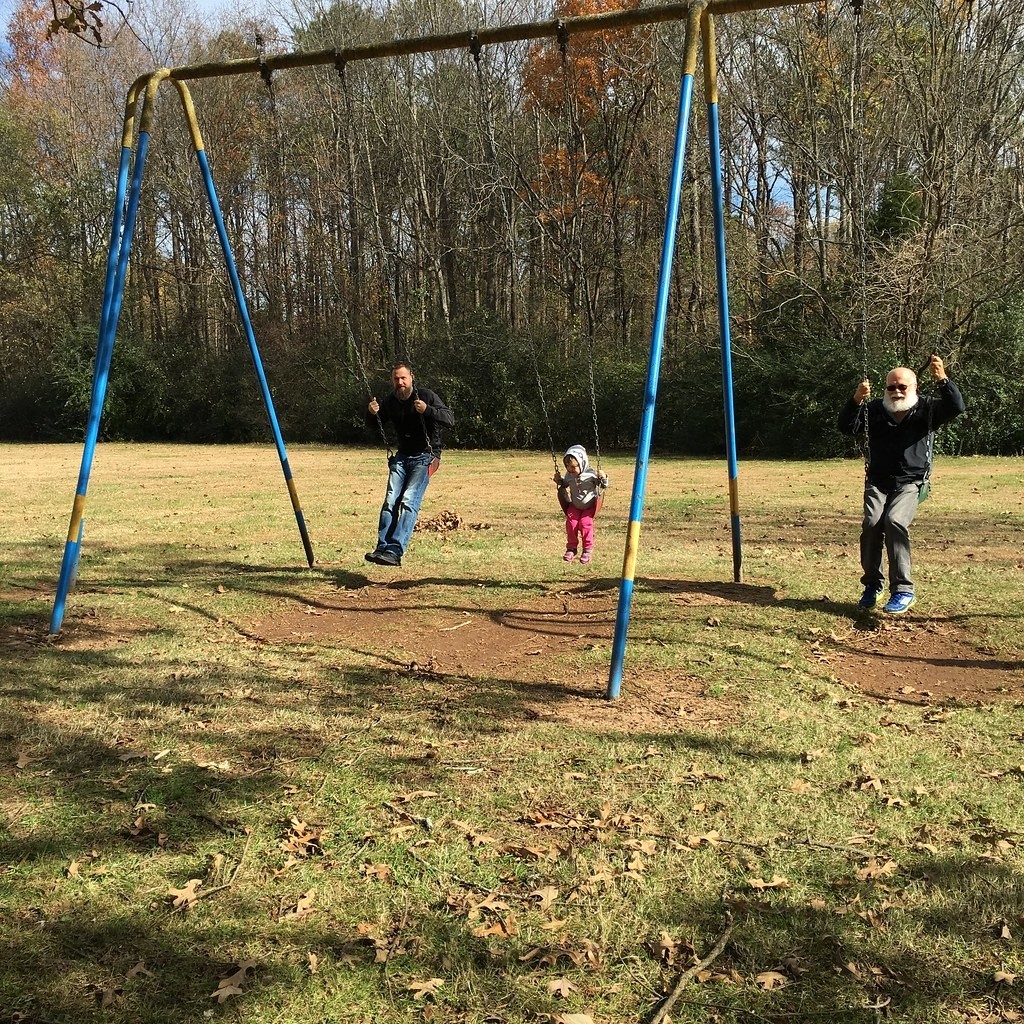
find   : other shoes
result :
[563,548,577,562]
[579,548,594,564]
[365,550,402,566]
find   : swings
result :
[257,56,442,478]
[472,33,610,514]
[849,0,972,502]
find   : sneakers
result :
[883,591,917,614]
[858,584,885,610]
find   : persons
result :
[553,445,608,564]
[836,354,965,615]
[364,364,454,567]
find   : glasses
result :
[886,383,913,391]
